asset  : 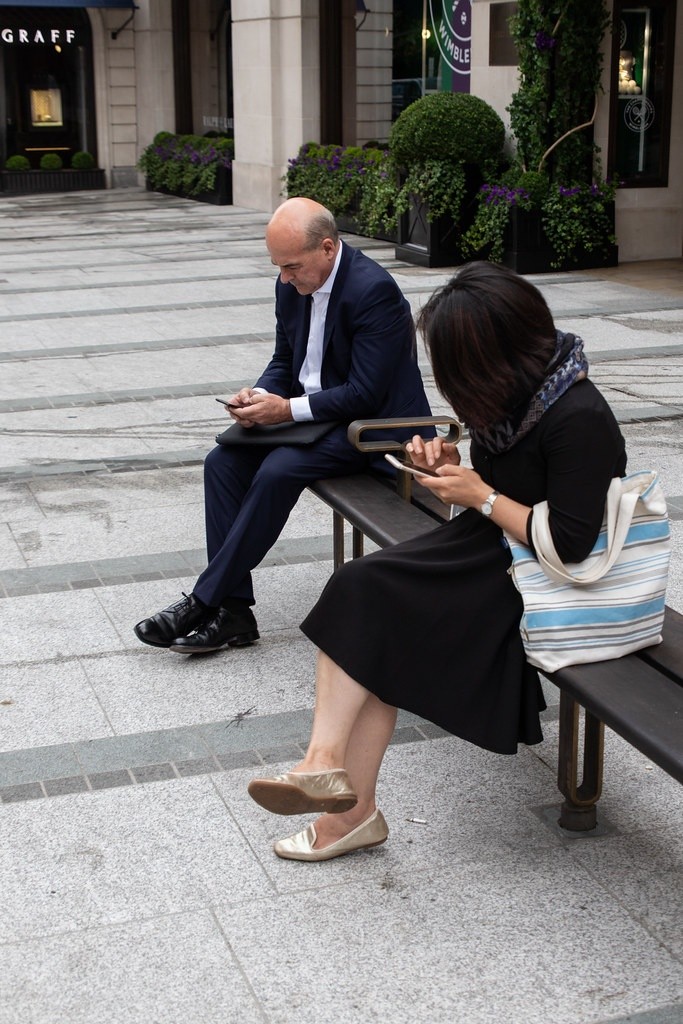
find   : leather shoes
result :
[134,593,212,648]
[169,607,260,654]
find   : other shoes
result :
[275,808,389,861]
[247,769,358,815]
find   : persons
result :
[133,196,438,655]
[247,263,629,862]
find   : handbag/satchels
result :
[503,470,664,673]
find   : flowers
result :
[137,132,234,193]
[282,142,402,233]
[462,175,618,269]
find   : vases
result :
[144,157,233,206]
[309,184,402,244]
[486,203,616,276]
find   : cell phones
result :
[383,453,444,480]
[215,397,246,408]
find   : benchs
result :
[309,416,683,832]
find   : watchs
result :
[481,490,501,518]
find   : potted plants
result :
[0,152,105,196]
[389,91,503,268]
